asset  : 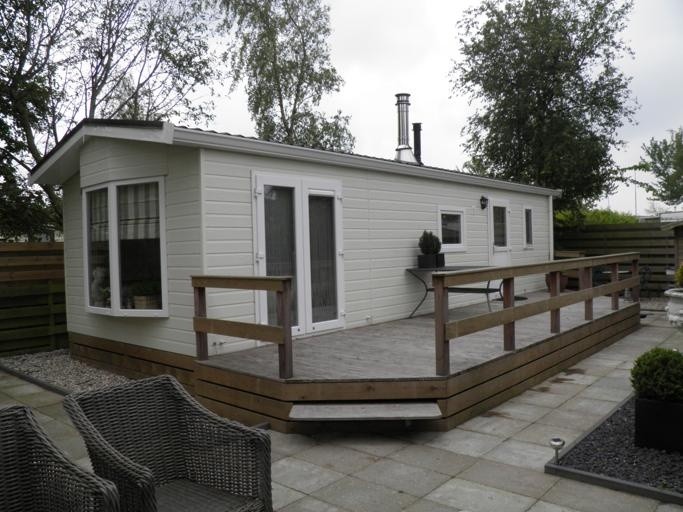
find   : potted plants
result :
[664,263,683,327]
[128,283,161,309]
[631,348,682,452]
[92,269,110,307]
[418,231,444,268]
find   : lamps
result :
[480,195,488,209]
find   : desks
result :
[405,266,506,319]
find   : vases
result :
[546,274,569,292]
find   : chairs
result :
[64,374,272,512]
[0,404,120,512]
